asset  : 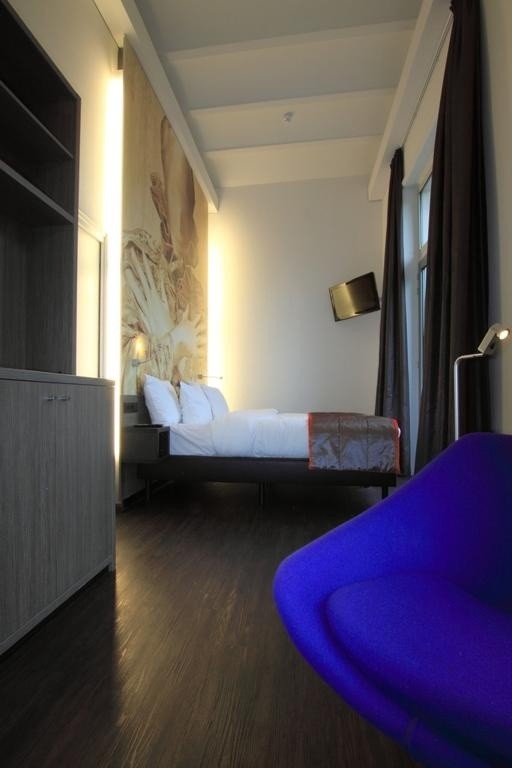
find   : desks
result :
[121,424,171,464]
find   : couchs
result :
[272,431,512,767]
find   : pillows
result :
[142,375,230,426]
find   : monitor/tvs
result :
[329,272,381,322]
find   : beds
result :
[138,408,401,507]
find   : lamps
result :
[453,323,509,442]
[131,333,163,427]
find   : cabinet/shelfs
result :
[1,0,114,654]
